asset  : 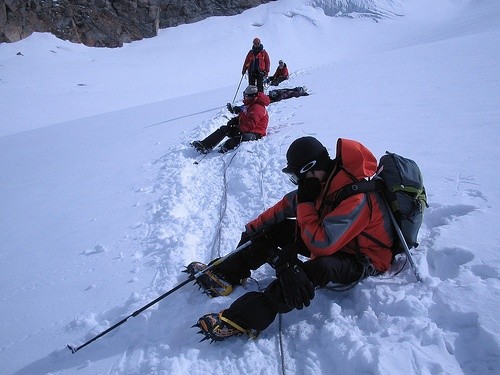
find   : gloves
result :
[226,102,235,113]
[297,177,322,204]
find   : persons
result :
[192,38,290,155]
[180,137,393,344]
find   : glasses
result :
[254,43,260,45]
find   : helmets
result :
[243,85,258,95]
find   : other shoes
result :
[193,140,209,154]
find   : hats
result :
[281,136,332,172]
[253,38,260,43]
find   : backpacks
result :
[324,150,430,254]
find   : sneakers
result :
[190,310,248,344]
[182,262,233,298]
